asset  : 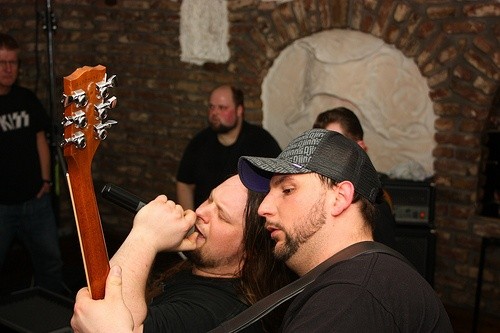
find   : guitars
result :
[56,65,118,300]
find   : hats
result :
[238,129,384,206]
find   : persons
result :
[110,173,286,333]
[69,128,453,333]
[312,107,392,205]
[173,84,284,210]
[0,32,64,296]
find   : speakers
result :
[391,228,436,282]
[0,283,75,333]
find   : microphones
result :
[101,183,195,238]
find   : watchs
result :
[43,179,53,187]
